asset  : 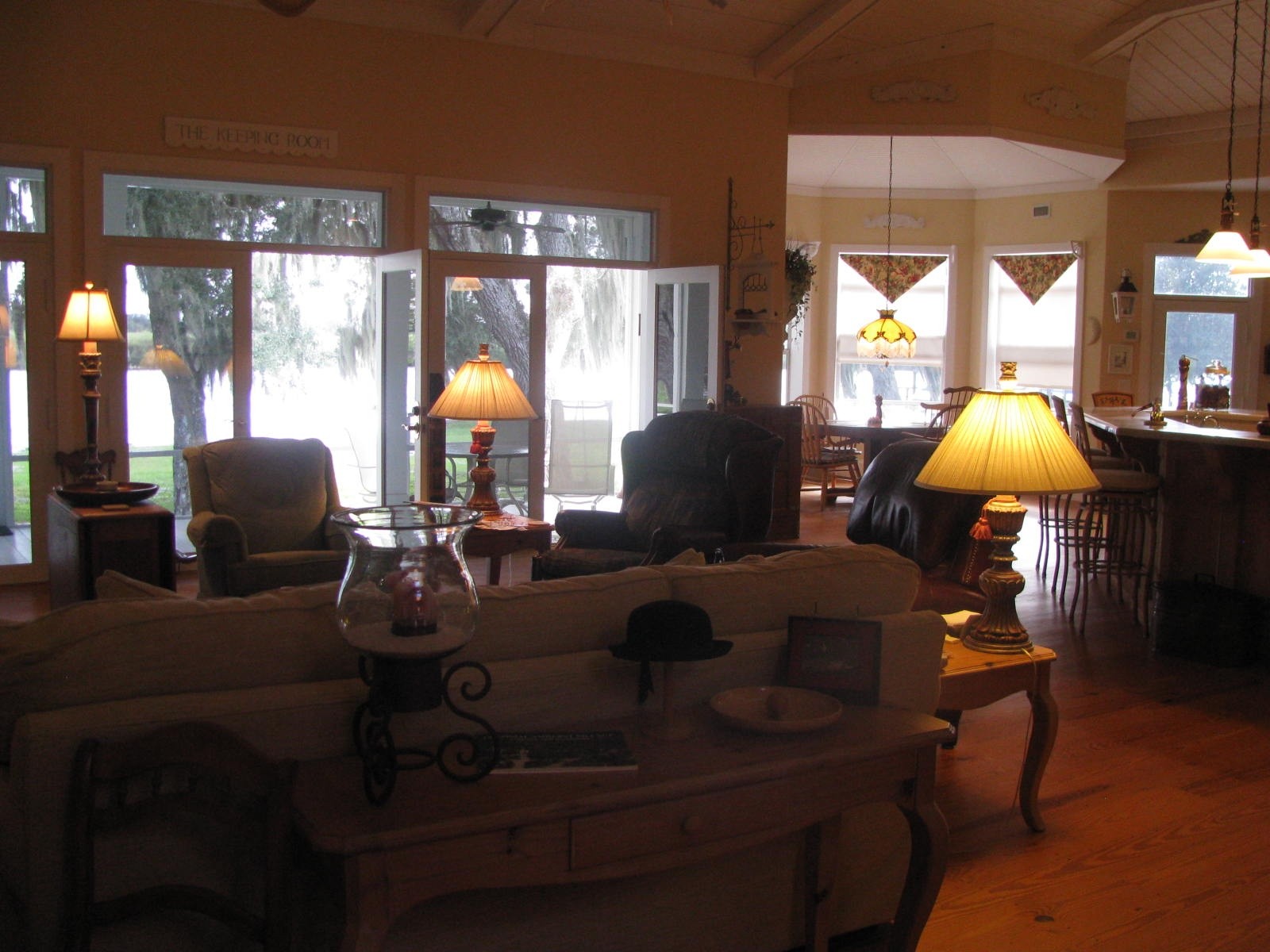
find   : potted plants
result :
[734,308,753,328]
[786,232,817,349]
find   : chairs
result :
[784,386,982,511]
[56,448,116,485]
[66,722,300,952]
[544,399,615,512]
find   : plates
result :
[52,481,158,505]
[709,685,843,734]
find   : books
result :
[478,728,639,775]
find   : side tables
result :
[463,513,556,586]
[938,610,1059,832]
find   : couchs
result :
[0,410,1021,952]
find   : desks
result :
[295,706,958,952]
[46,494,176,613]
[804,420,946,475]
[445,442,529,518]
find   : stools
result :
[1040,391,1160,639]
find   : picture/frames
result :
[1108,344,1133,375]
[786,616,882,708]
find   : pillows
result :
[627,476,724,548]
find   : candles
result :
[394,576,439,626]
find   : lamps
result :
[52,282,127,483]
[428,345,537,516]
[1111,268,1139,323]
[855,137,917,360]
[451,277,482,291]
[915,361,1102,653]
[1194,0,1270,278]
[141,344,190,379]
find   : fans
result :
[431,201,566,235]
